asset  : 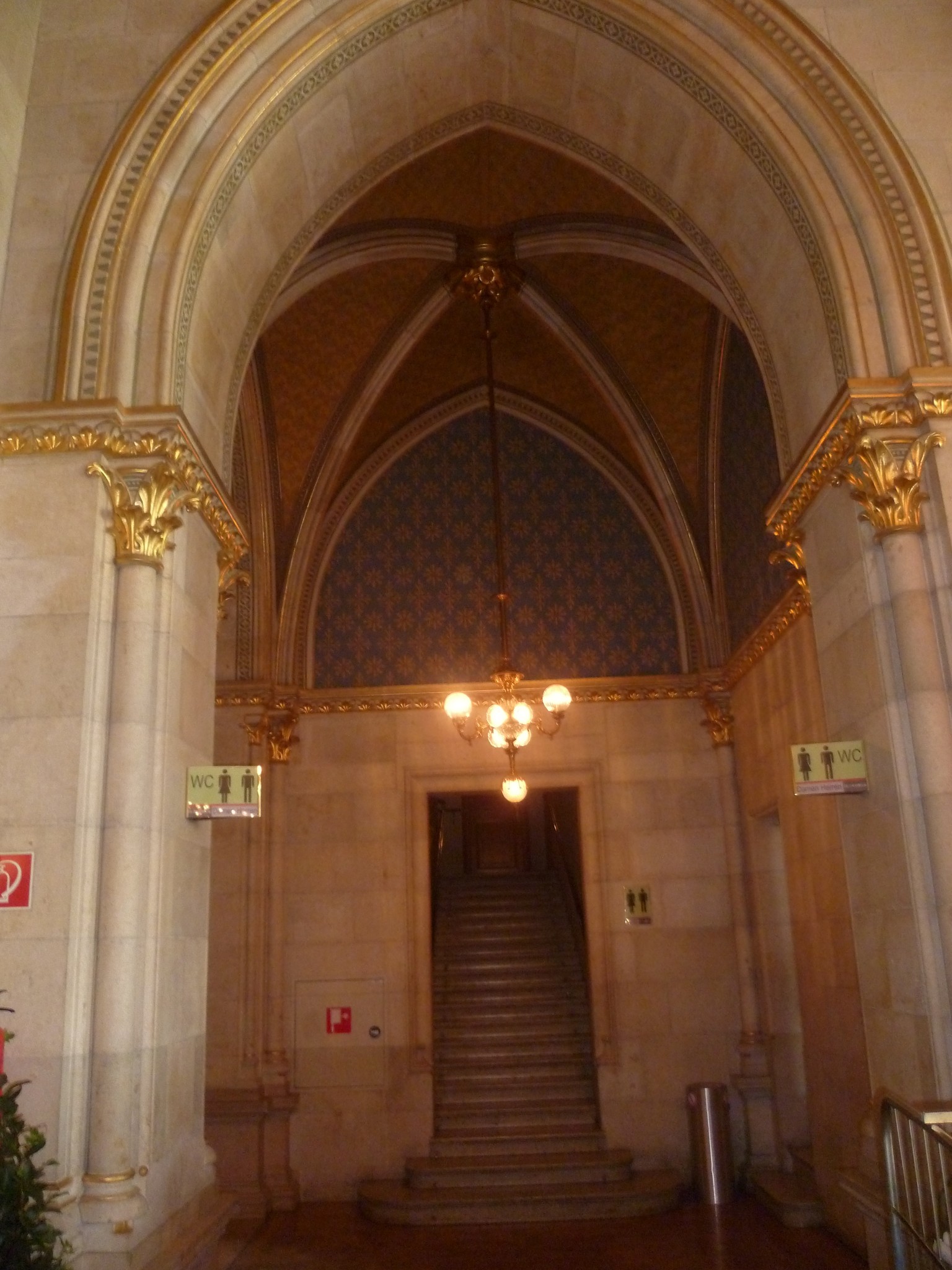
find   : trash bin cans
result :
[685,1080,735,1206]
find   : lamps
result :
[443,238,571,806]
